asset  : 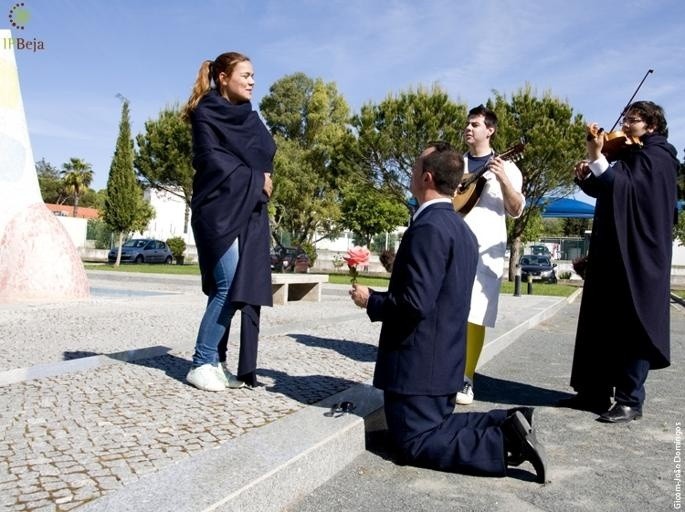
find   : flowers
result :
[343,247,370,287]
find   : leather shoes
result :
[558,395,610,411]
[600,403,642,423]
[505,408,548,482]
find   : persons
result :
[179,52,277,392]
[349,142,548,483]
[451,104,526,405]
[570,101,679,423]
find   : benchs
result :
[270,273,329,305]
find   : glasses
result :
[331,402,357,418]
[619,118,644,126]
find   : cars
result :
[529,245,552,258]
[268,246,310,275]
[106,238,173,265]
[516,254,558,285]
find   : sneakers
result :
[456,381,475,405]
[217,363,245,388]
[186,364,225,392]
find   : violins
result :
[585,128,641,161]
